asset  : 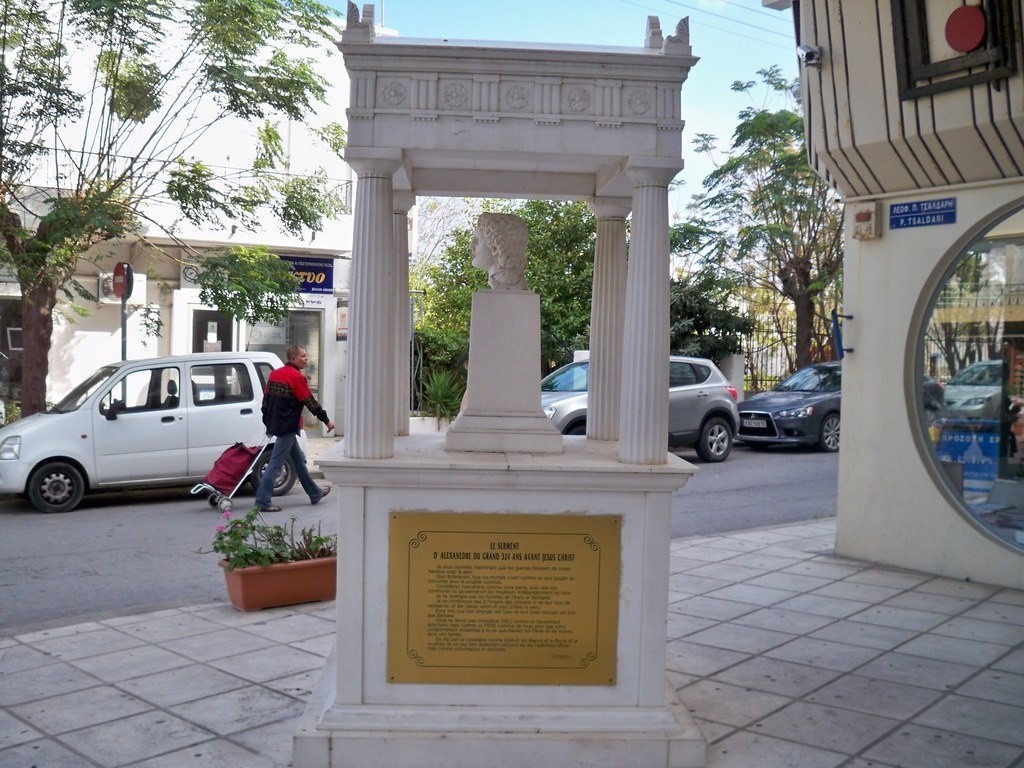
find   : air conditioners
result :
[97,273,147,304]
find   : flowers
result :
[194,504,337,571]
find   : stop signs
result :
[113,262,126,297]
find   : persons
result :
[469,212,529,291]
[255,344,335,512]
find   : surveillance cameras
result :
[796,45,818,62]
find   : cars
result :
[944,359,1003,428]
[734,361,945,452]
[541,356,740,463]
[0,351,310,513]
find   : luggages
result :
[191,434,273,513]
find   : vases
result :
[218,550,338,613]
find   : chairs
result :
[163,380,179,408]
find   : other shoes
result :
[311,486,330,504]
[261,506,281,511]
